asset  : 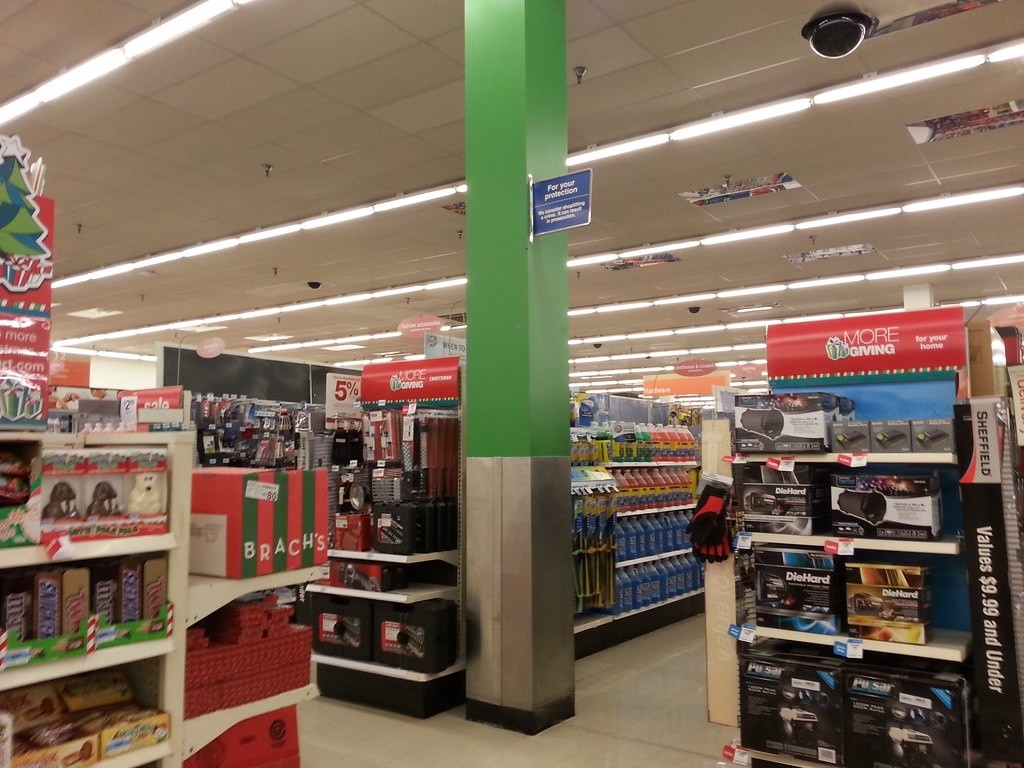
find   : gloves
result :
[685,484,735,565]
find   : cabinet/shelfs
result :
[570,393,705,661]
[304,358,466,719]
[721,392,974,768]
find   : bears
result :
[128,472,160,514]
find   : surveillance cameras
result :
[307,282,320,289]
[594,344,601,348]
[800,11,873,59]
[688,307,701,314]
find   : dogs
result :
[43,482,81,520]
[87,481,125,517]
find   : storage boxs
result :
[373,601,457,674]
[734,393,856,453]
[184,594,312,768]
[752,546,843,637]
[738,638,842,766]
[829,474,944,542]
[310,594,372,661]
[829,418,955,453]
[732,461,827,536]
[844,561,933,645]
[0,430,42,548]
[10,722,100,768]
[41,450,169,546]
[73,705,171,762]
[842,652,972,768]
[188,467,329,580]
